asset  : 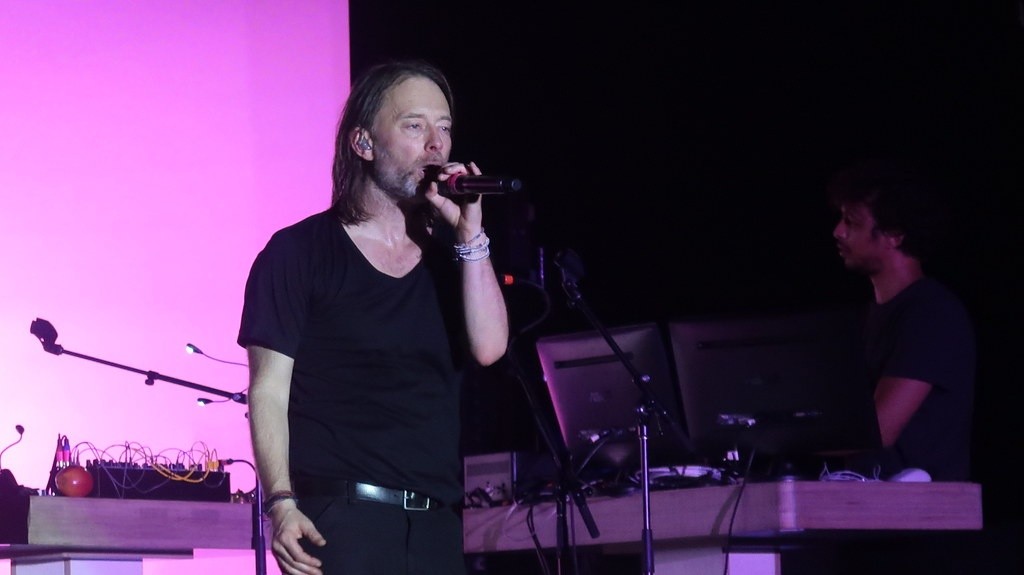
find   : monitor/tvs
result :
[538,302,887,498]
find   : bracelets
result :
[264,491,298,515]
[453,228,490,263]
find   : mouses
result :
[892,468,932,484]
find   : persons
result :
[738,163,975,482]
[236,57,509,575]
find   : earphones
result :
[357,138,372,151]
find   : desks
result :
[0,492,274,575]
[463,482,983,575]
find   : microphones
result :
[436,173,522,199]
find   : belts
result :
[348,482,440,512]
